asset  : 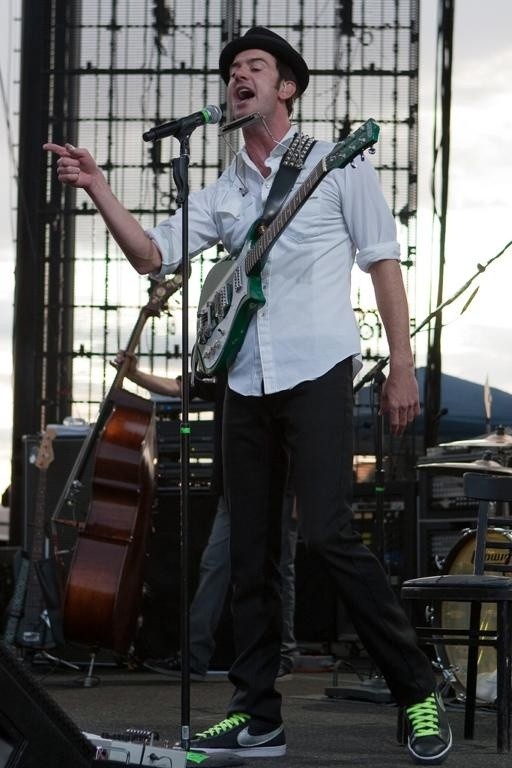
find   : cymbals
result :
[416,459,510,481]
[438,435,512,452]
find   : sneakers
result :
[405,686,453,760]
[143,653,206,680]
[175,714,288,758]
[275,663,293,681]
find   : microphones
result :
[141,102,222,143]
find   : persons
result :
[108,351,301,686]
[41,27,452,763]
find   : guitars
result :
[198,119,381,379]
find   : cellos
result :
[43,255,191,654]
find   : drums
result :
[425,526,509,707]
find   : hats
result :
[221,27,310,101]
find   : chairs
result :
[396,471,511,753]
